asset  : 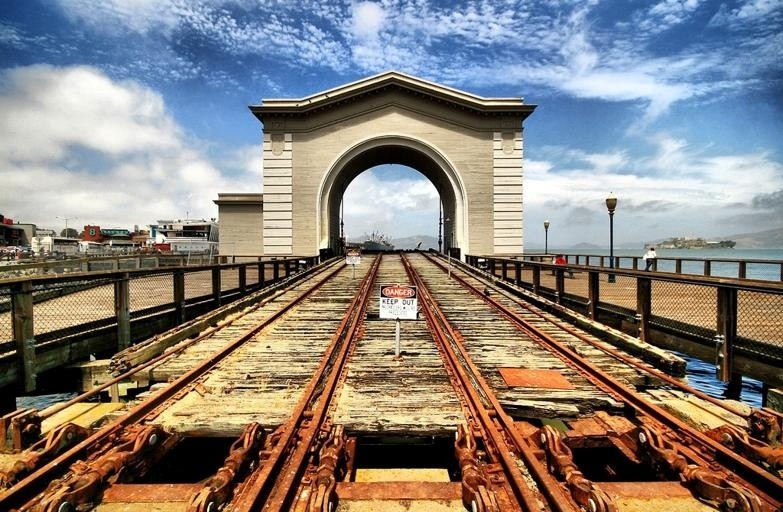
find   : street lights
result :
[55,216,77,238]
[605,192,617,284]
[543,219,550,255]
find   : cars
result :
[0,246,32,255]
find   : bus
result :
[78,241,108,258]
[30,236,80,260]
[103,241,140,254]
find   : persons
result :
[11,249,37,260]
[643,248,657,272]
[556,254,570,270]
